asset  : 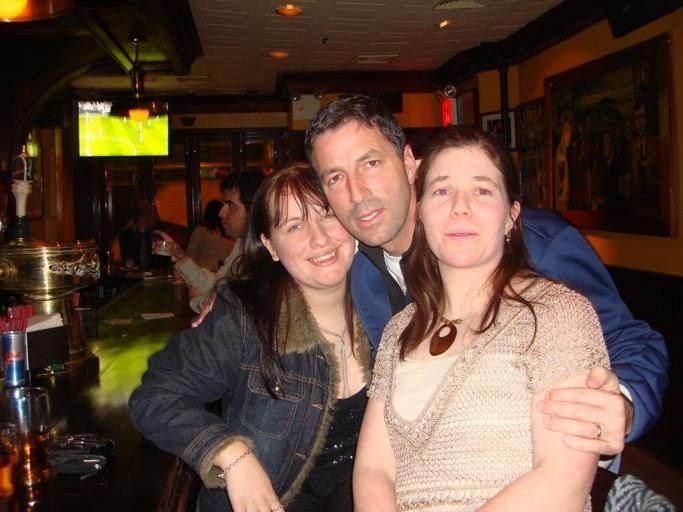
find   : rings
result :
[592,422,603,441]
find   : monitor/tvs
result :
[85,324,178,412]
[73,96,174,161]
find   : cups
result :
[151,240,174,273]
[7,386,51,451]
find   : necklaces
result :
[317,313,350,399]
[429,313,472,355]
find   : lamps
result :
[124,34,152,126]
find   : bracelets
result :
[218,448,256,480]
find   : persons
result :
[567,111,605,211]
[352,123,611,510]
[126,157,379,512]
[617,81,660,221]
[118,167,264,316]
[303,94,676,511]
